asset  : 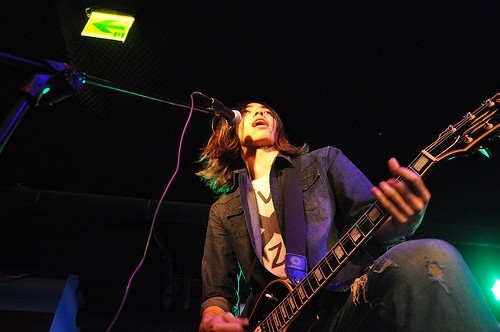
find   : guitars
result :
[242,92,500,332]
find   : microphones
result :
[200,94,242,126]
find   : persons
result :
[195,100,500,332]
